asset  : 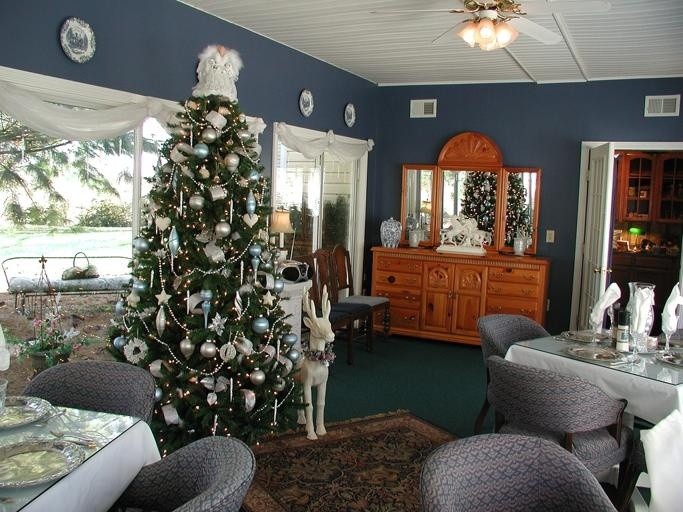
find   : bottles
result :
[616,324,629,352]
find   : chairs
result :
[419,434,620,511]
[333,244,390,341]
[475,313,550,434]
[110,435,257,511]
[485,354,634,499]
[20,361,156,426]
[288,256,351,375]
[309,249,370,364]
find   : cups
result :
[658,313,680,353]
[587,305,604,347]
[0,379,8,414]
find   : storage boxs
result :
[369,132,549,346]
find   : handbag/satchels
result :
[61,252,99,280]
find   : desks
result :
[612,252,681,308]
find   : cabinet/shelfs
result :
[615,153,683,222]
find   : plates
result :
[561,331,609,343]
[655,352,683,367]
[565,346,625,362]
[0,439,86,488]
[0,396,51,430]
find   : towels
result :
[590,283,621,324]
[662,282,683,332]
[634,288,654,334]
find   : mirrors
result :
[406,169,432,241]
[504,172,535,245]
[442,171,496,245]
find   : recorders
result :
[275,261,313,283]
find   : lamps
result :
[450,1,527,51]
[268,209,296,260]
[628,228,643,252]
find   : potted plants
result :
[105,43,304,459]
[27,258,70,375]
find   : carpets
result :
[240,409,451,512]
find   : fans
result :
[370,0,612,47]
